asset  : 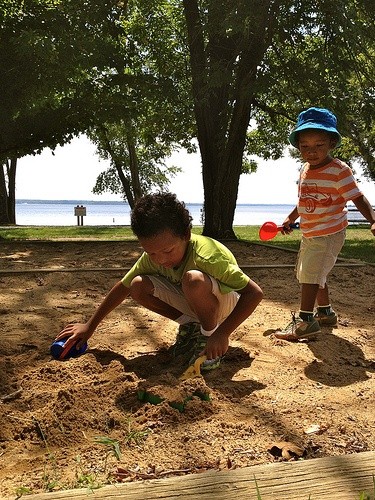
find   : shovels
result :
[258,221,300,241]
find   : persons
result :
[55,192,263,373]
[275,107,375,339]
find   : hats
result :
[289,107,342,147]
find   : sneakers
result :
[169,322,201,359]
[313,306,337,327]
[275,311,322,341]
[188,334,225,371]
